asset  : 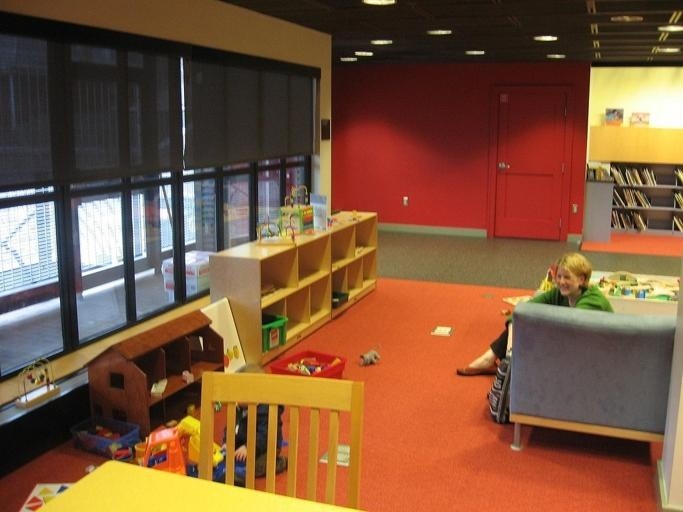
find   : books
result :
[586,164,683,231]
[318,445,351,468]
[429,325,453,337]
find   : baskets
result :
[332,291,349,309]
[68,417,141,458]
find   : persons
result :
[219,362,288,479]
[454,251,614,377]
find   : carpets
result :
[0,278,664,512]
[580,233,683,257]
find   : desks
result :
[29,457,361,512]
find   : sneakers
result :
[457,365,499,376]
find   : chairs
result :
[197,371,364,510]
[509,300,677,452]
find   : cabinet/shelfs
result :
[86,326,225,439]
[208,209,379,368]
[609,161,683,235]
